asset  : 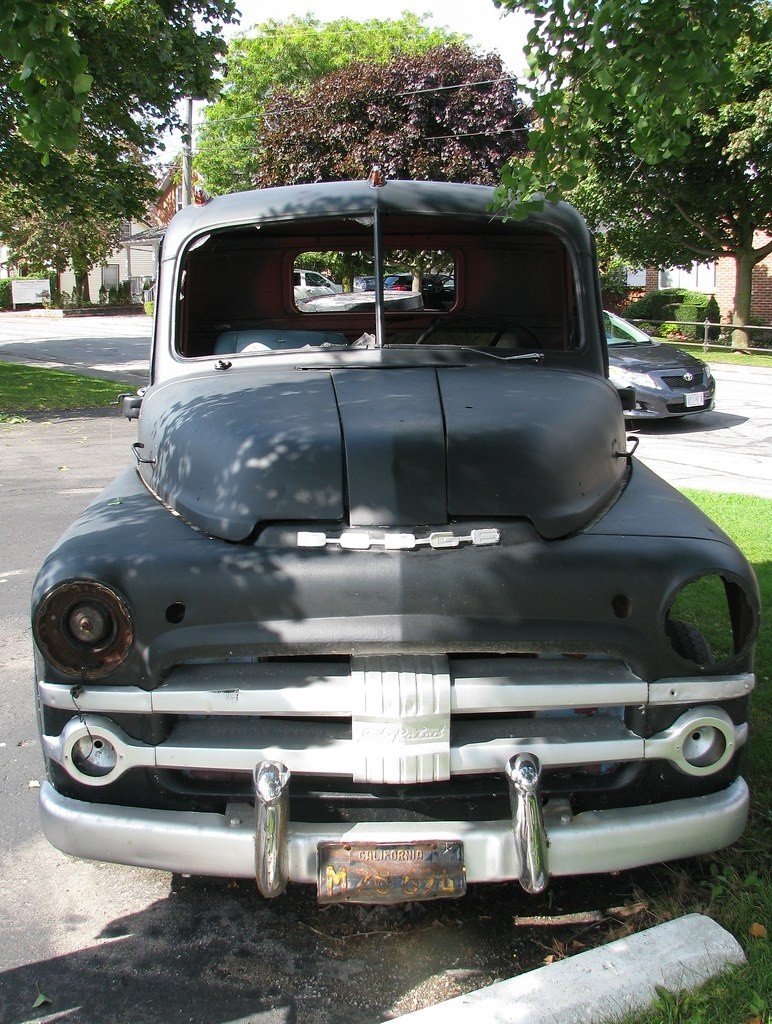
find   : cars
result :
[32,168,758,907]
[601,309,715,429]
[294,269,342,300]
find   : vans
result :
[383,273,454,301]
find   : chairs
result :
[391,330,522,347]
[215,330,348,354]
